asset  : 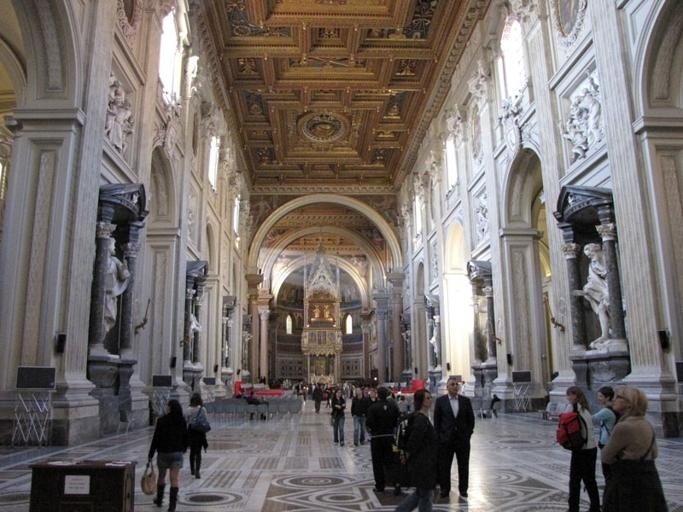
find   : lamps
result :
[346,316,352,336]
[286,315,293,336]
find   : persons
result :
[148,399,188,512]
[432,377,474,498]
[601,386,669,512]
[312,382,322,413]
[591,386,619,450]
[258,395,269,421]
[183,392,211,478]
[100,225,128,342]
[247,392,257,420]
[343,381,354,399]
[491,395,500,418]
[365,386,399,493]
[263,376,266,385]
[393,389,438,512]
[324,390,331,408]
[582,243,626,349]
[296,382,312,401]
[427,371,436,387]
[564,386,601,512]
[351,388,366,446]
[260,376,263,383]
[330,388,345,447]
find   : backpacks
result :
[556,413,588,451]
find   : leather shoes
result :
[460,489,468,497]
[439,490,450,498]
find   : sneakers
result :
[335,439,345,447]
[354,440,365,446]
[373,485,385,492]
[394,484,401,495]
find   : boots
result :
[167,487,178,511]
[151,484,166,505]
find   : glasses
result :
[450,383,458,386]
[424,396,434,401]
[612,394,628,401]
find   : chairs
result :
[197,391,307,424]
[416,380,494,419]
[117,409,137,436]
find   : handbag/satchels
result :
[330,414,339,426]
[188,423,208,448]
[142,459,158,495]
